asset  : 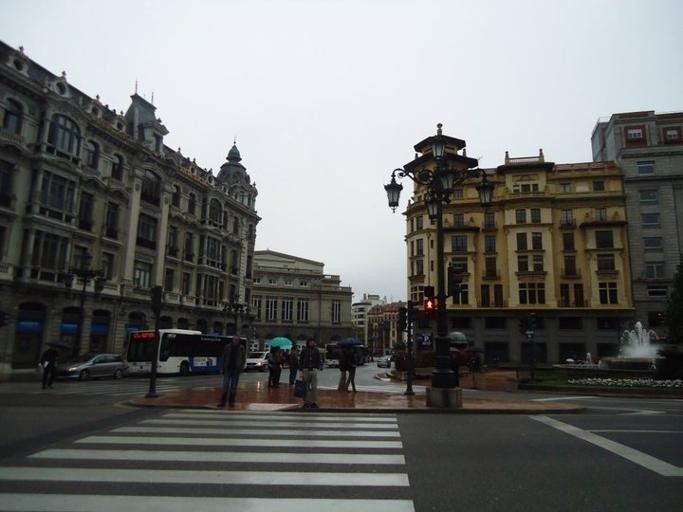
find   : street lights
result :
[223,293,250,334]
[64,253,105,359]
[383,123,496,408]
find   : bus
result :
[123,327,250,375]
[325,343,366,366]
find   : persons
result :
[344,346,358,392]
[335,345,357,391]
[298,338,321,405]
[468,353,480,389]
[266,345,298,389]
[214,336,245,410]
[39,344,59,390]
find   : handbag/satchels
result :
[294,380,306,398]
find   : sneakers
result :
[217,402,234,409]
[336,387,357,393]
[303,403,318,409]
[268,383,280,388]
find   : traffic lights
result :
[448,266,464,297]
[424,299,435,312]
[149,286,163,316]
[519,310,538,340]
[399,301,419,330]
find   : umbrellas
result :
[463,345,487,356]
[44,340,73,354]
[266,336,292,348]
[336,337,362,352]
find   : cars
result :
[244,350,270,372]
[377,356,391,369]
[55,351,130,382]
